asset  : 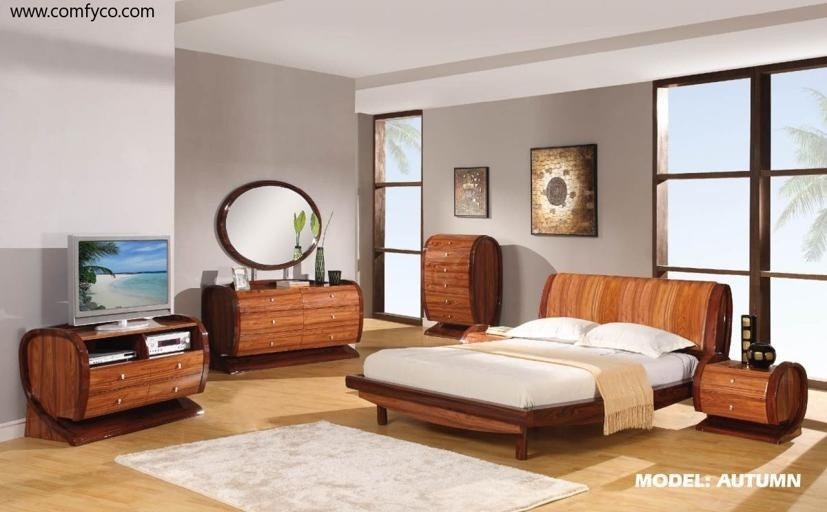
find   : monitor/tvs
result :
[67,232,176,332]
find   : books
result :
[276,279,311,288]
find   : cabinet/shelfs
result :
[420,233,504,340]
[200,277,365,375]
[17,314,212,447]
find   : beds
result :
[345,271,734,462]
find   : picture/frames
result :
[530,143,599,237]
[453,166,489,218]
[231,267,251,291]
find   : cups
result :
[328,271,341,286]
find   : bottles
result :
[746,341,777,369]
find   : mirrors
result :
[216,179,322,271]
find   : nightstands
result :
[456,324,514,343]
[691,351,809,446]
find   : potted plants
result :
[310,209,334,285]
[293,210,307,260]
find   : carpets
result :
[111,418,589,512]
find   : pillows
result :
[502,317,699,360]
